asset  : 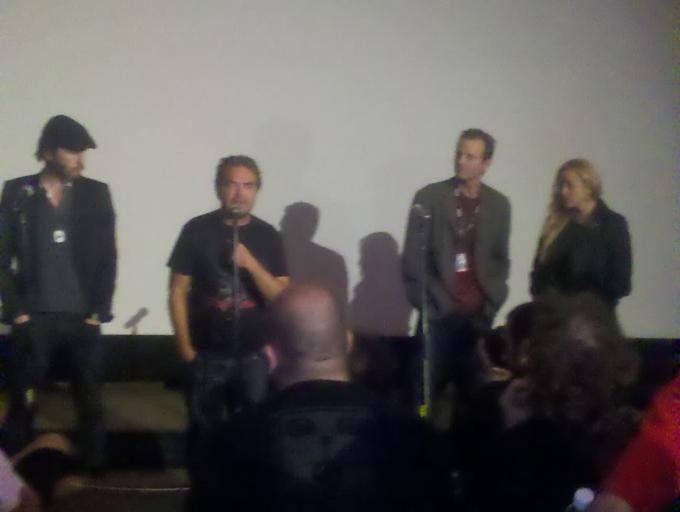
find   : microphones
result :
[412,203,432,219]
[12,184,34,212]
[229,207,242,230]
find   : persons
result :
[1,390,71,510]
[163,155,290,511]
[435,294,680,511]
[2,116,121,473]
[211,288,454,512]
[400,128,512,437]
[530,157,633,314]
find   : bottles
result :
[568,485,595,512]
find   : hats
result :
[42,113,96,152]
